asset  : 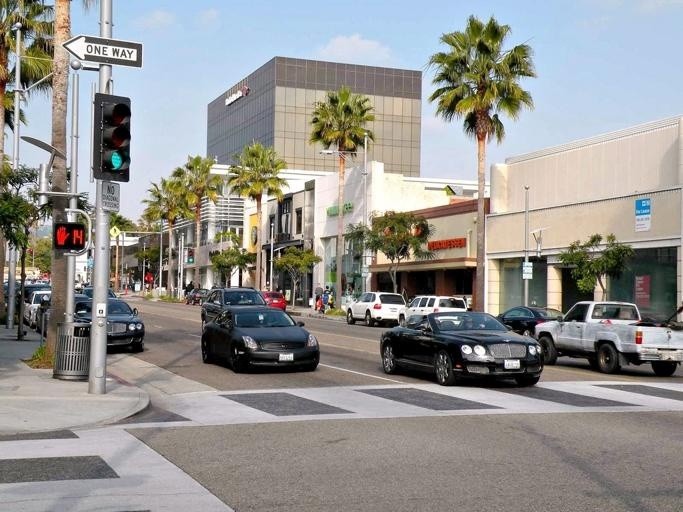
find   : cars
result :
[3,275,144,353]
[184,288,209,306]
[494,306,563,336]
[259,290,285,312]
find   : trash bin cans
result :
[53,322,92,380]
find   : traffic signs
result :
[62,35,144,69]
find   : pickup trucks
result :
[535,300,683,376]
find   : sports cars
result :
[200,304,319,373]
[379,312,543,385]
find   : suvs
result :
[347,292,406,327]
[200,287,267,332]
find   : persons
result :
[316,286,334,314]
[186,281,194,295]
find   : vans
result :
[398,296,468,325]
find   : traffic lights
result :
[186,247,194,264]
[93,92,132,183]
[52,221,86,251]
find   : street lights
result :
[269,222,286,291]
[318,133,368,292]
[521,184,550,305]
